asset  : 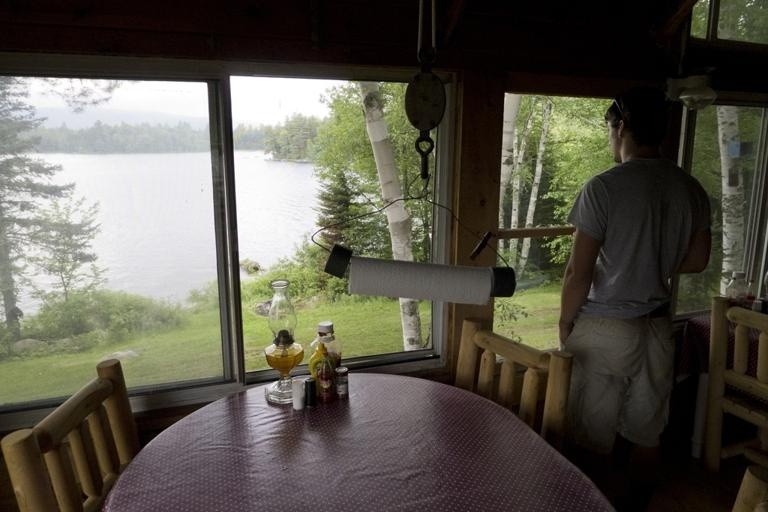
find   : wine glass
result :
[262,278,304,405]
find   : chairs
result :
[729,466,767,512]
[449,317,574,444]
[702,298,767,473]
[0,355,142,512]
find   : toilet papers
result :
[347,256,492,306]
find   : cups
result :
[752,300,761,312]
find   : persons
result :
[558,98,712,512]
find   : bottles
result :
[305,376,320,408]
[748,280,754,298]
[725,270,748,309]
[308,341,339,396]
[291,378,305,411]
[310,321,342,368]
[316,362,334,403]
[334,366,350,400]
